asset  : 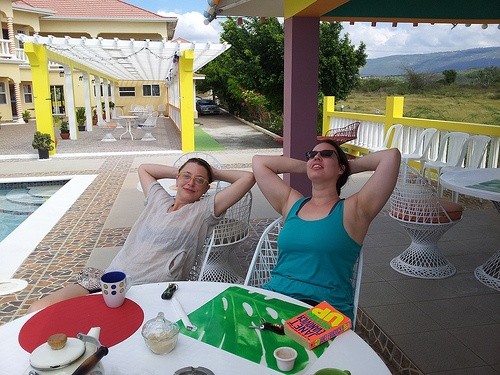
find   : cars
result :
[196,100,219,115]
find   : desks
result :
[118,116,139,140]
[439,168,500,202]
[0,281,391,375]
[129,111,147,128]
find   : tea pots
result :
[24,327,108,375]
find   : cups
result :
[100,271,131,308]
[313,368,351,375]
[274,347,297,372]
[174,366,215,375]
[142,312,180,353]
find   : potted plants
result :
[22,110,30,122]
[31,132,54,159]
[92,101,115,125]
[76,107,87,131]
[59,121,70,139]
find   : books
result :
[284,300,352,349]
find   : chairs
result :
[182,121,500,329]
[99,104,167,142]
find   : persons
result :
[27,158,256,313]
[253,140,401,332]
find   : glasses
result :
[305,150,338,162]
[178,171,209,186]
